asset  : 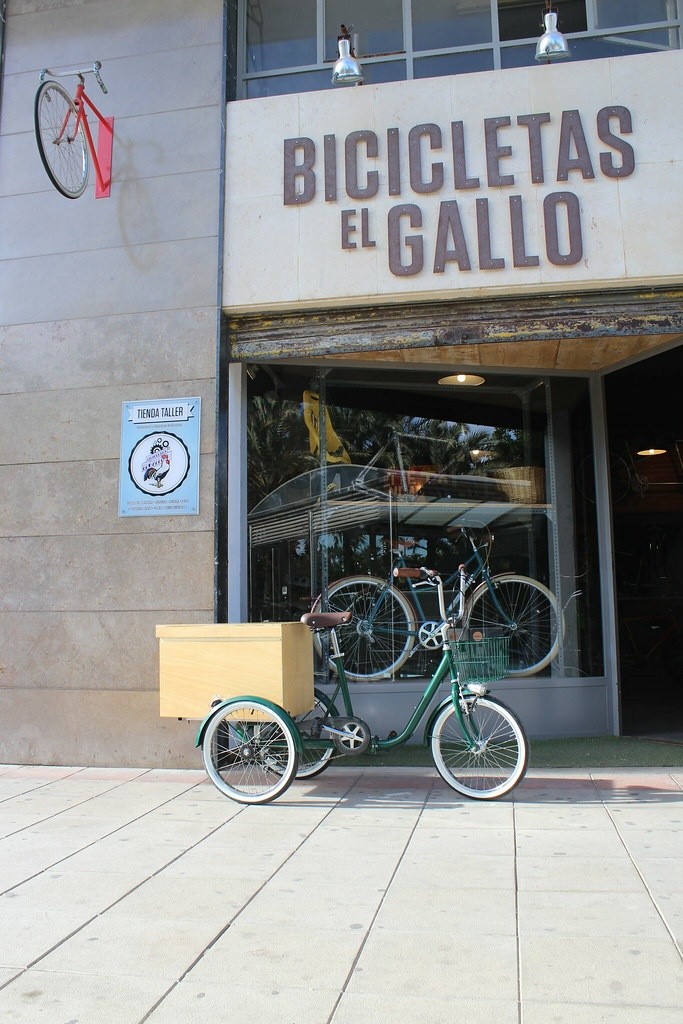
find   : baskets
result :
[450,636,512,685]
[484,466,539,503]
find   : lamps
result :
[330,24,366,85]
[534,0,569,63]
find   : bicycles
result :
[308,521,566,683]
[557,527,683,596]
[583,606,683,682]
[577,431,683,505]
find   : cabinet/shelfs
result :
[248,363,564,680]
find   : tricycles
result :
[194,564,530,801]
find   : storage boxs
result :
[155,621,314,722]
